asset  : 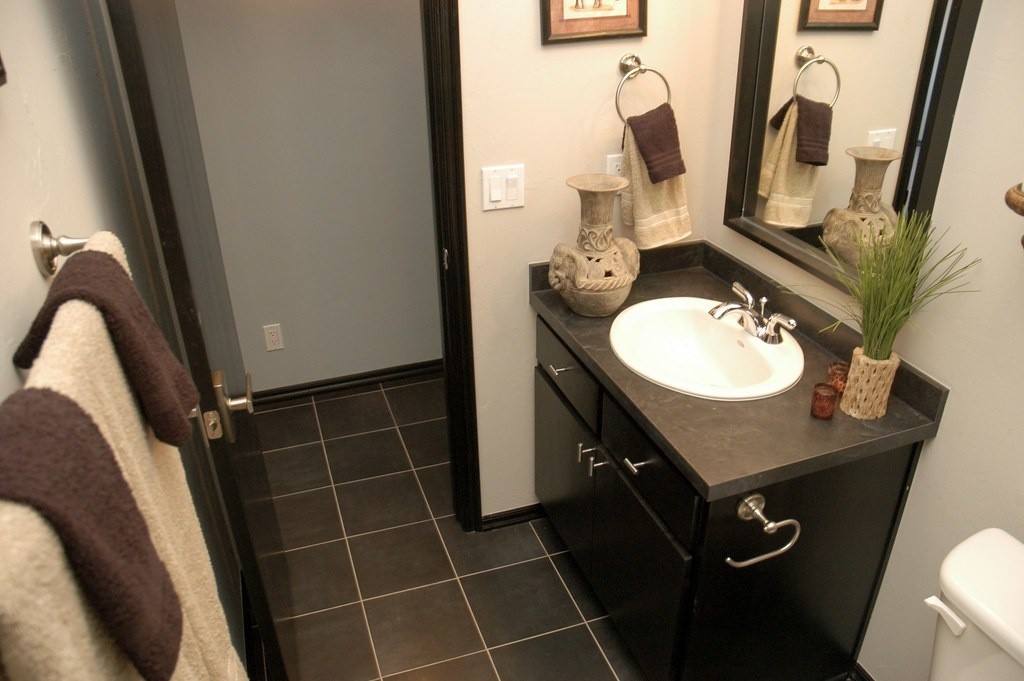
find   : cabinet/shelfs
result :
[534,313,916,680]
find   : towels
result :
[13,247,200,448]
[621,102,686,185]
[770,93,832,166]
[758,100,832,229]
[2,228,255,681]
[621,117,691,250]
[1,388,184,680]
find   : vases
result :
[547,172,639,318]
[821,146,903,270]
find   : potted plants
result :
[776,207,984,420]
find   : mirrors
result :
[722,0,985,323]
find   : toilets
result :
[923,523,1023,681]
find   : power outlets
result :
[606,152,624,194]
[264,323,284,351]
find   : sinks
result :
[608,295,805,400]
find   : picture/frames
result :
[540,0,648,45]
[797,0,884,31]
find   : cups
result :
[826,361,851,395]
[812,383,840,420]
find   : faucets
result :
[708,299,764,336]
